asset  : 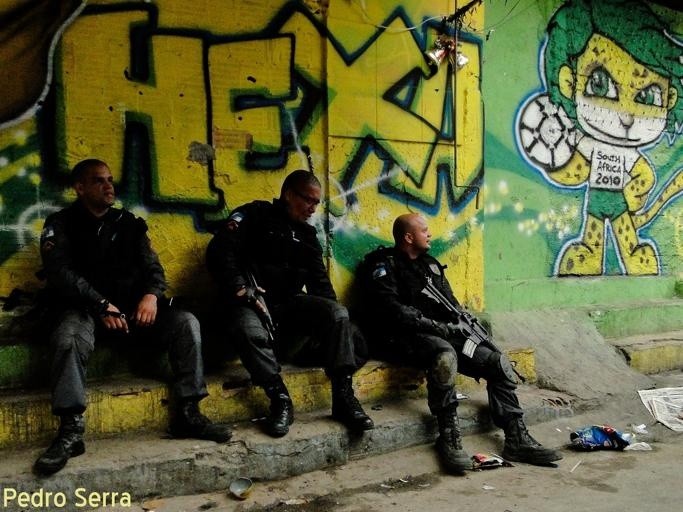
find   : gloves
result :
[418,317,449,339]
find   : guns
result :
[2,288,127,322]
[242,267,279,341]
[420,281,527,383]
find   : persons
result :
[349,211,562,471]
[35,158,233,473]
[206,170,374,440]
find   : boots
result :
[502,413,563,463]
[260,373,294,438]
[32,413,85,476]
[168,399,233,443]
[332,372,374,430]
[435,410,473,470]
